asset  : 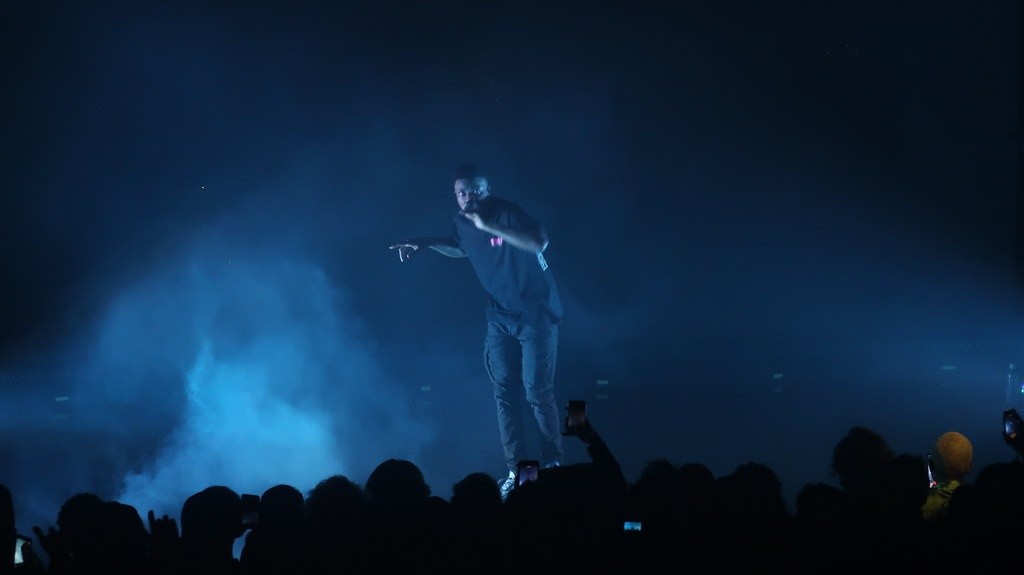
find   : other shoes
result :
[544,461,560,468]
[500,470,524,503]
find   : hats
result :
[453,472,501,510]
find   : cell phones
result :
[242,494,259,528]
[13,536,32,565]
[518,461,539,485]
[1003,410,1018,436]
[567,401,586,428]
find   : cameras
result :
[623,519,643,536]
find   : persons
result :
[389,164,562,495]
[0,409,1024,575]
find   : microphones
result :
[464,202,480,213]
